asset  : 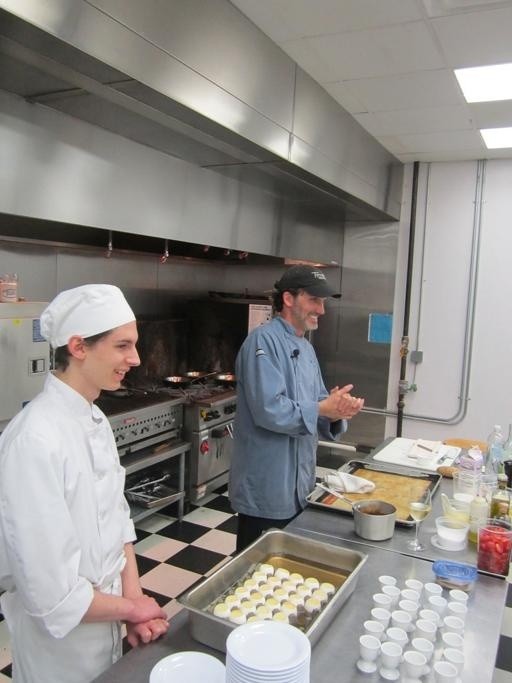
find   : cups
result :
[475,518,512,577]
[356,574,470,683]
[451,472,476,501]
[435,516,470,547]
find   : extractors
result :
[1,209,295,266]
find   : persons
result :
[0,282,169,682]
[229,262,365,553]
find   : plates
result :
[147,650,224,682]
[224,620,312,683]
[430,534,468,552]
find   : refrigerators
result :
[1,301,55,438]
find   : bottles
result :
[490,474,512,520]
[467,496,490,542]
[475,424,511,497]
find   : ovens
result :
[187,418,236,487]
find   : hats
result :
[39,283,136,348]
[274,264,342,301]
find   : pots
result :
[161,372,217,388]
[184,370,213,381]
[213,372,235,385]
[314,482,398,543]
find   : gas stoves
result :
[140,382,236,431]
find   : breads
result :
[214,564,335,625]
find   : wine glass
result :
[406,486,432,552]
